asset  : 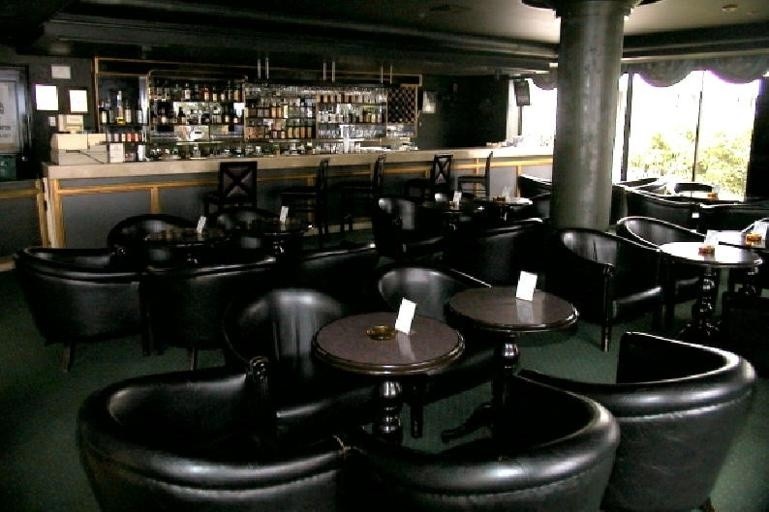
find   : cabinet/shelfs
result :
[97,66,422,142]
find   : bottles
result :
[99,77,147,158]
[244,78,416,158]
[148,79,245,157]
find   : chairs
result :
[74,356,347,512]
[523,329,769,511]
[346,372,621,510]
